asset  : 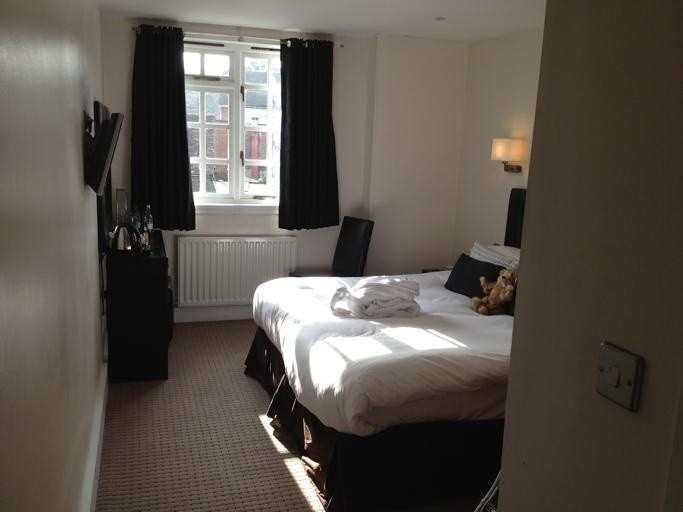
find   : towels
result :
[330,270,420,321]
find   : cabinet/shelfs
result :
[103,229,174,382]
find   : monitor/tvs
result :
[83,112,124,196]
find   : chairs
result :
[290,214,374,278]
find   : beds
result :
[251,189,523,512]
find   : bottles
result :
[142,205,153,233]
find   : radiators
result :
[174,234,298,309]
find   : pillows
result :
[442,238,521,309]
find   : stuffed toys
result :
[471,267,517,313]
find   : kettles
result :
[112,223,141,258]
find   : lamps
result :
[490,138,526,173]
